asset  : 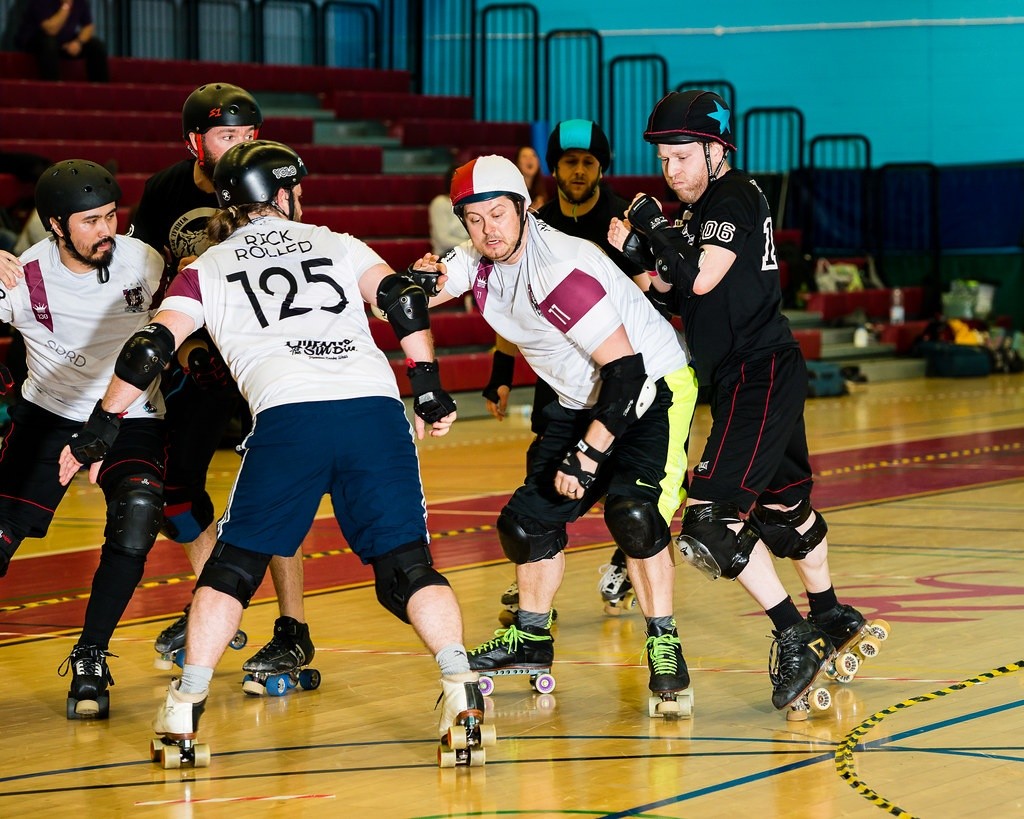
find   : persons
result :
[413,155,692,697]
[430,92,674,607]
[59,141,484,745]
[607,89,868,711]
[0,84,316,697]
[0,0,111,83]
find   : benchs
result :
[0,59,974,398]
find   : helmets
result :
[644,90,738,155]
[182,83,264,137]
[546,120,610,177]
[36,159,121,231]
[212,140,307,207]
[449,155,533,222]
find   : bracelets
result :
[74,37,83,47]
[60,3,73,11]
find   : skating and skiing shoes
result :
[809,607,890,684]
[243,616,320,697]
[56,643,120,720]
[500,586,559,628]
[465,622,557,697]
[598,549,638,618]
[769,620,856,720]
[436,671,498,769]
[152,601,248,669]
[149,678,214,772]
[641,620,696,718]
